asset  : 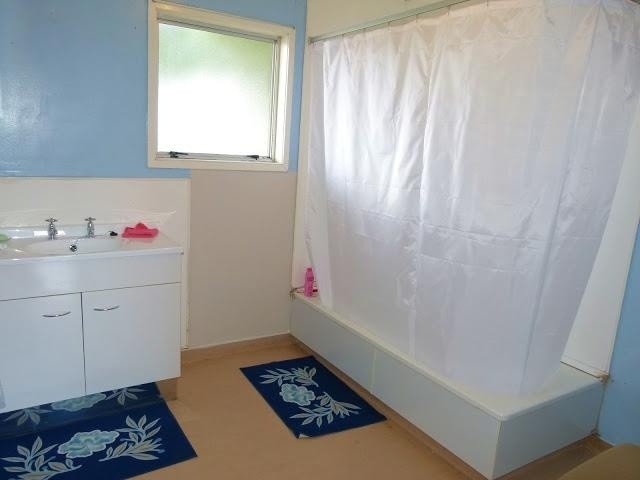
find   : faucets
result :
[45,219,58,240]
[85,218,96,238]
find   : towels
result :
[123,222,158,238]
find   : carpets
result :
[0,382,198,480]
[239,355,388,440]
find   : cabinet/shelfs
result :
[0,253,181,414]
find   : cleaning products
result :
[304,268,314,296]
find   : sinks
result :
[25,237,132,255]
